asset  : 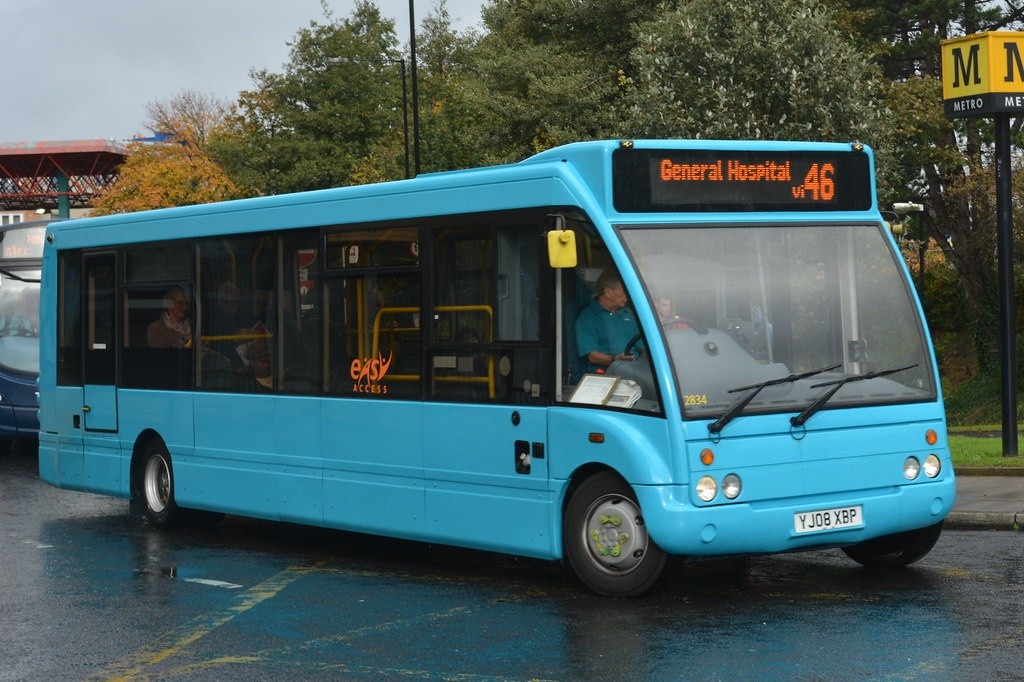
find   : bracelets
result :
[612,354,616,362]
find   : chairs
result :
[393,343,421,374]
[549,268,593,384]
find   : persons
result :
[237,342,273,393]
[147,287,192,347]
[575,266,646,385]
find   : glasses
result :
[172,300,189,307]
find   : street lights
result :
[326,56,410,178]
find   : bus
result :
[34,138,958,598]
[0,218,82,439]
